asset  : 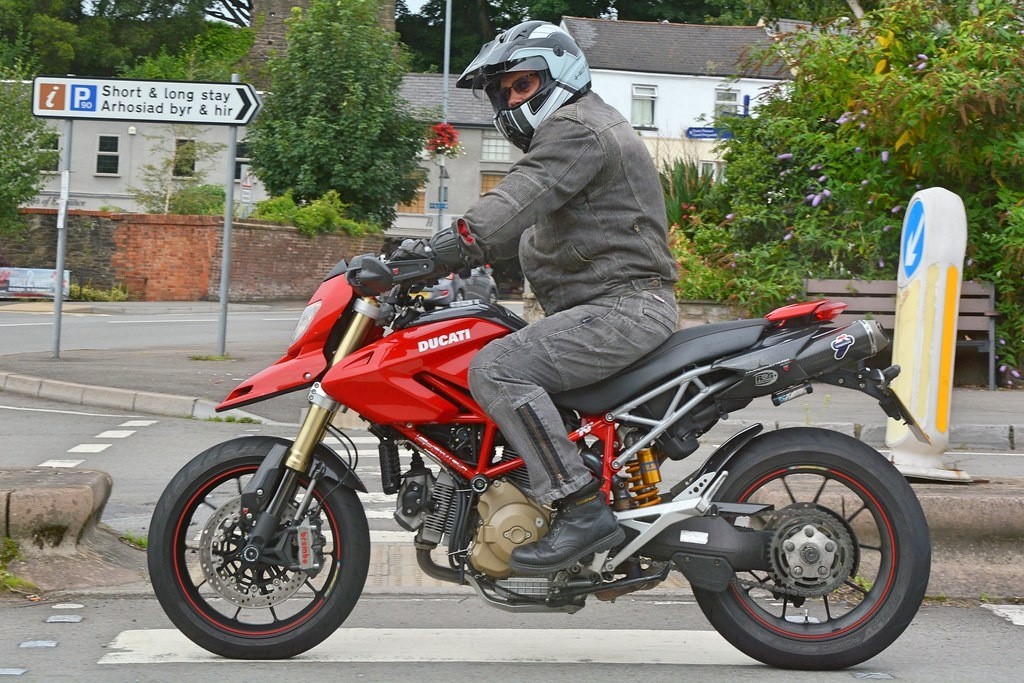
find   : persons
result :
[381,18,679,568]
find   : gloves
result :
[389,227,471,294]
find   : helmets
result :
[455,20,592,153]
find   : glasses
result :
[498,73,538,105]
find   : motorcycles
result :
[147,241,933,671]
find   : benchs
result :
[802,278,996,392]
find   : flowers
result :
[429,123,467,159]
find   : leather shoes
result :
[509,491,626,574]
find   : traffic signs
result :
[31,76,263,126]
[685,126,737,139]
